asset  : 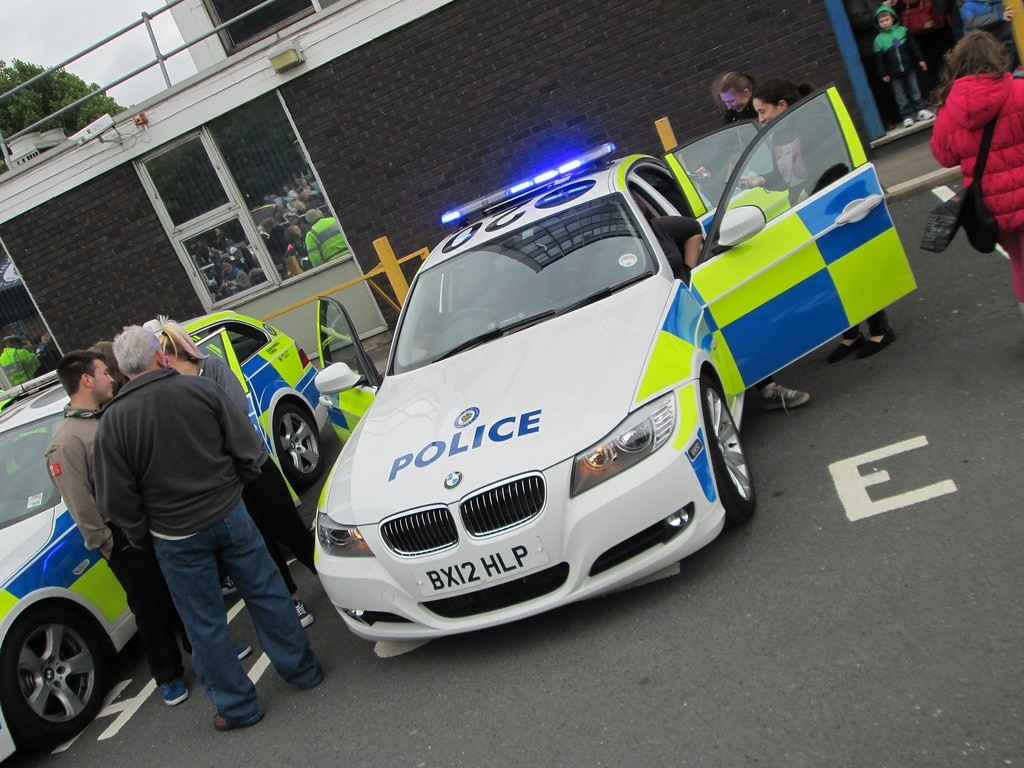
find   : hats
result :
[283,208,301,221]
[3,336,25,341]
[230,247,239,255]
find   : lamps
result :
[266,40,306,74]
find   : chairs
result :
[6,432,52,499]
[445,266,530,327]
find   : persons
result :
[0,330,63,387]
[695,71,773,180]
[655,216,809,411]
[45,314,327,732]
[928,29,1024,315]
[186,177,351,304]
[874,0,1015,128]
[0,390,13,412]
[736,77,897,364]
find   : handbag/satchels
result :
[920,181,1002,254]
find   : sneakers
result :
[292,598,314,628]
[763,384,810,411]
[161,675,189,706]
[232,639,254,661]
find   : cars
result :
[313,84,916,643]
[1,301,331,768]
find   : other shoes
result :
[827,328,896,363]
[903,109,934,127]
[1011,69,1024,78]
[213,713,264,731]
